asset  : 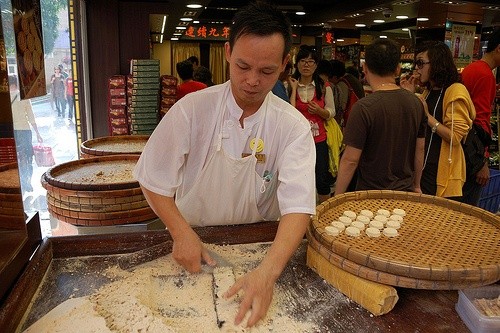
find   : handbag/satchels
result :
[336,79,359,122]
[324,116,344,177]
[33,141,55,167]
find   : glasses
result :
[300,59,314,65]
[415,60,428,70]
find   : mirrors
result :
[0,18,28,271]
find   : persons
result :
[460,31,500,206]
[272,62,292,104]
[132,4,316,327]
[57,64,69,79]
[317,59,366,128]
[176,56,216,102]
[335,38,426,196]
[8,75,44,193]
[285,46,335,204]
[400,40,476,198]
[51,65,65,118]
[63,69,75,121]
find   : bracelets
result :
[432,122,440,133]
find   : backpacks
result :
[459,120,492,190]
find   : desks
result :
[0,219,472,332]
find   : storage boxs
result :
[479,169,500,214]
